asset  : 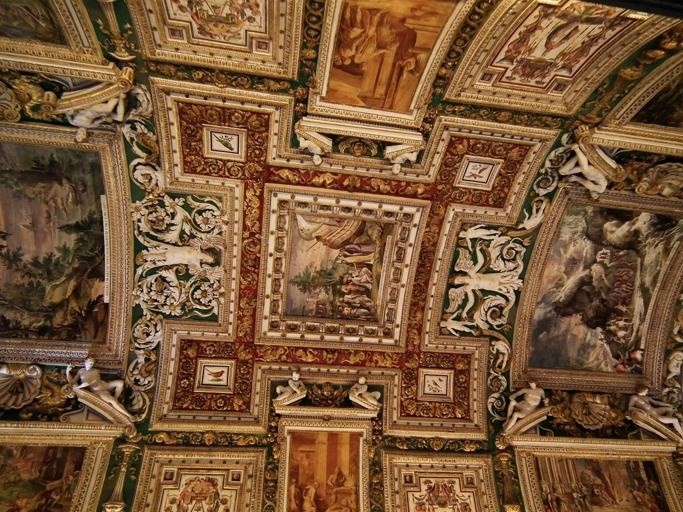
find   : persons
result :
[353,8,390,69]
[64,357,139,424]
[439,309,478,337]
[488,338,510,371]
[287,477,301,511]
[59,93,128,144]
[325,464,345,488]
[391,148,419,175]
[300,479,320,511]
[291,130,325,166]
[348,376,383,408]
[536,473,665,512]
[330,238,379,321]
[515,197,548,233]
[447,240,521,319]
[500,378,547,436]
[337,5,370,66]
[629,383,683,438]
[143,234,217,274]
[267,371,306,400]
[558,143,609,201]
